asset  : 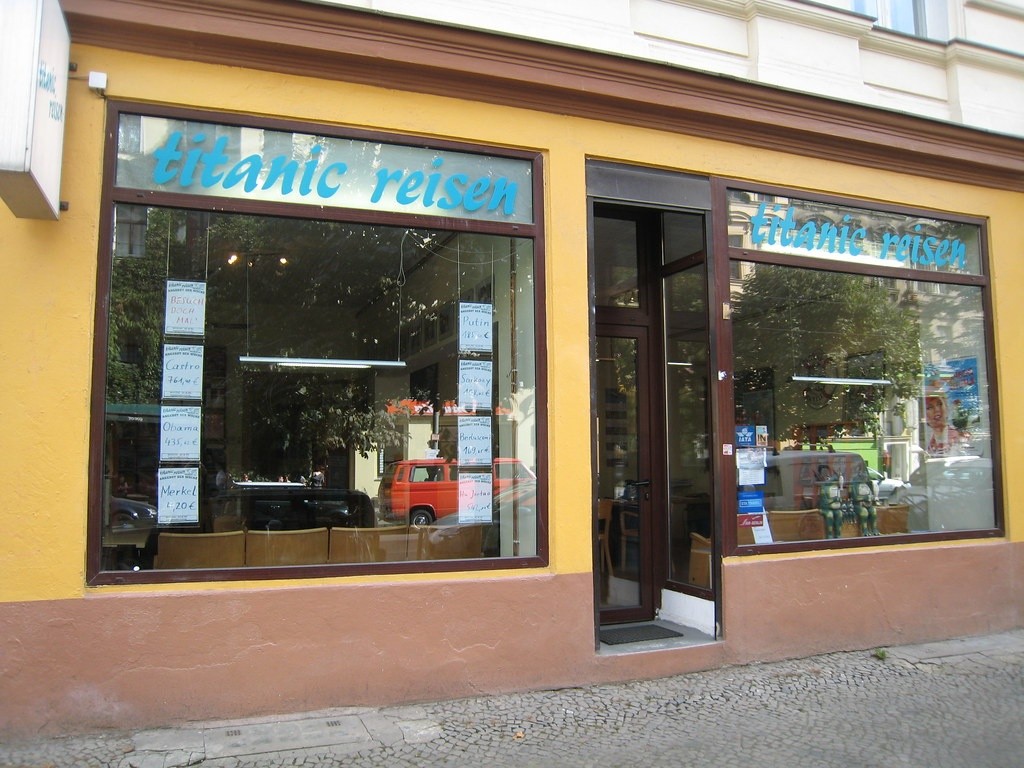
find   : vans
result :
[376,458,535,525]
[762,451,876,508]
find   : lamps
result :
[787,375,893,387]
[237,220,407,370]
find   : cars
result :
[866,467,911,503]
[108,495,159,524]
[902,453,994,529]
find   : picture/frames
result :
[384,274,497,360]
[841,348,888,419]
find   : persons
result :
[117,477,128,495]
[240,467,325,487]
[926,397,972,457]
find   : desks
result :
[611,497,639,570]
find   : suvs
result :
[204,489,377,530]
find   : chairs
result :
[598,498,614,576]
